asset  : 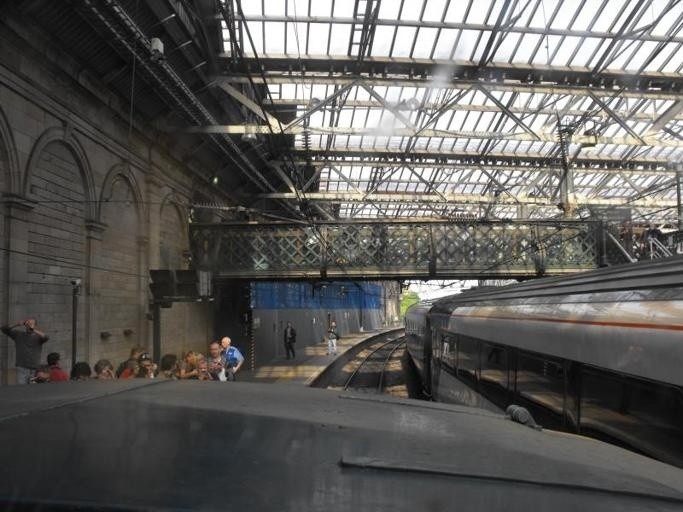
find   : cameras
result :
[34,376,45,384]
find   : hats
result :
[138,353,151,361]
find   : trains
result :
[405,254,683,469]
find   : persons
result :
[284,322,296,359]
[0,318,244,384]
[326,321,340,356]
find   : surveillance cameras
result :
[70,279,82,285]
